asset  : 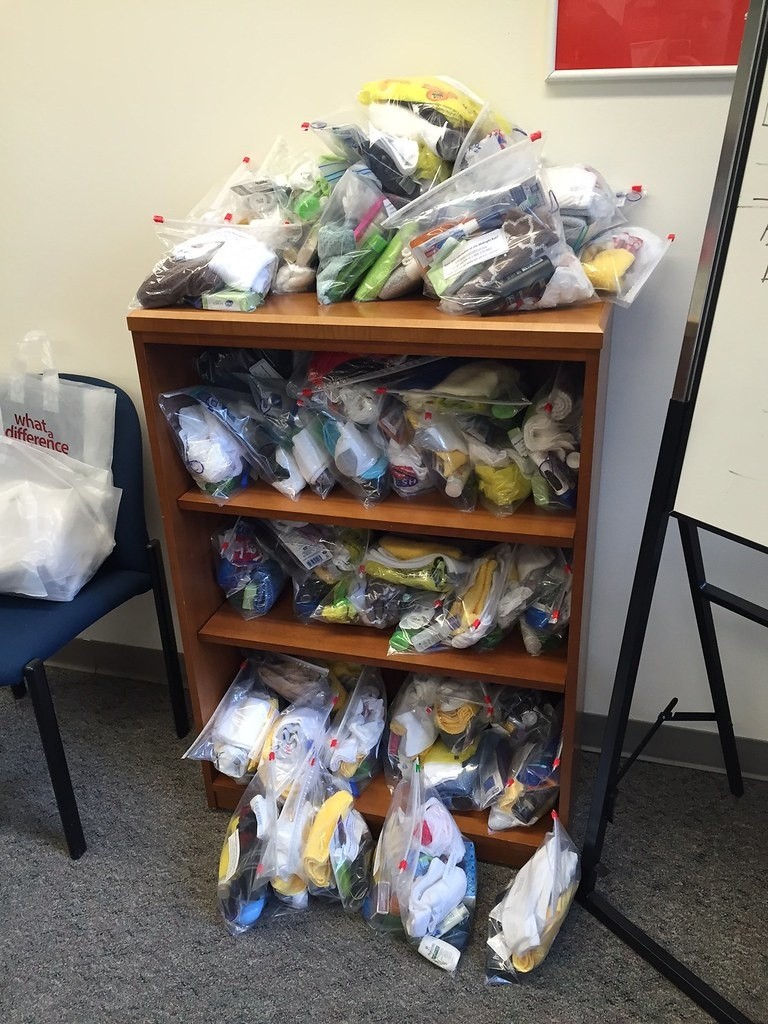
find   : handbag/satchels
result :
[0,331,122,602]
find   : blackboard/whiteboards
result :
[668,0,766,549]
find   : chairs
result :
[0,372,191,862]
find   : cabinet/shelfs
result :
[126,291,615,873]
